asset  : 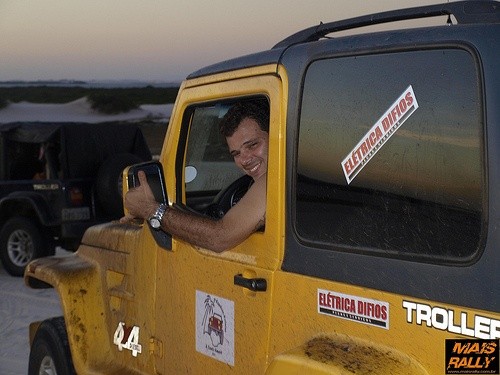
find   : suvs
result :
[0,120,153,277]
[26,0,500,371]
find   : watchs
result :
[150,203,167,231]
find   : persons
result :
[119,99,270,254]
